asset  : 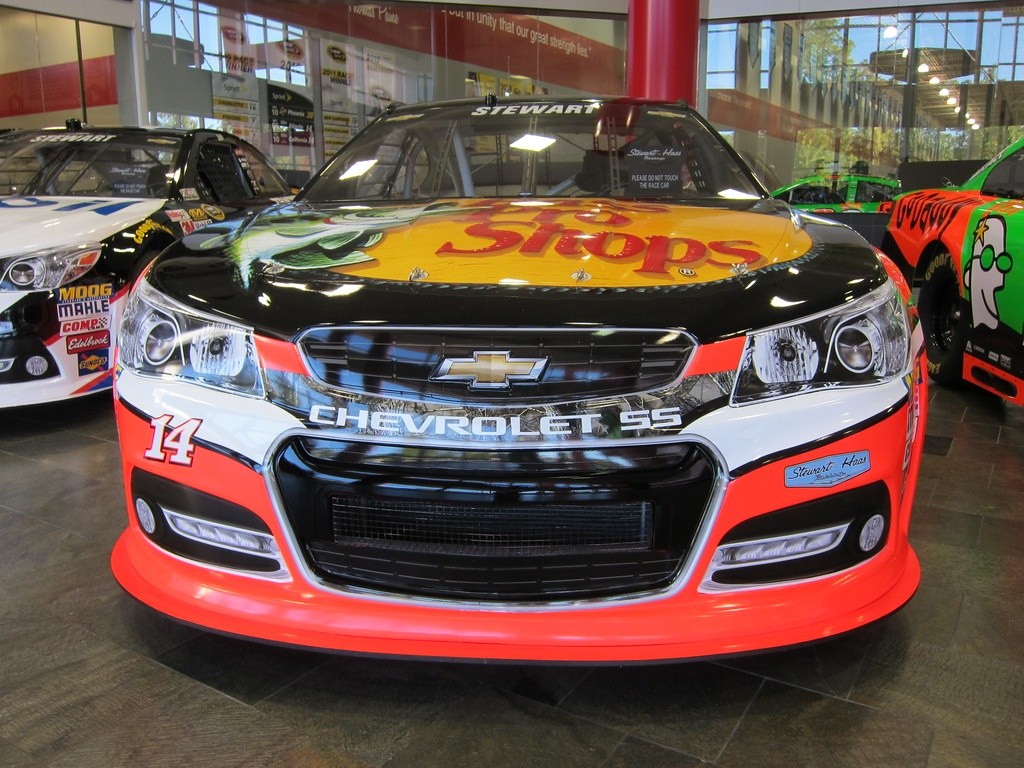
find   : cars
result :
[773,172,910,218]
[883,137,1024,423]
[110,96,931,662]
[0,116,305,412]
